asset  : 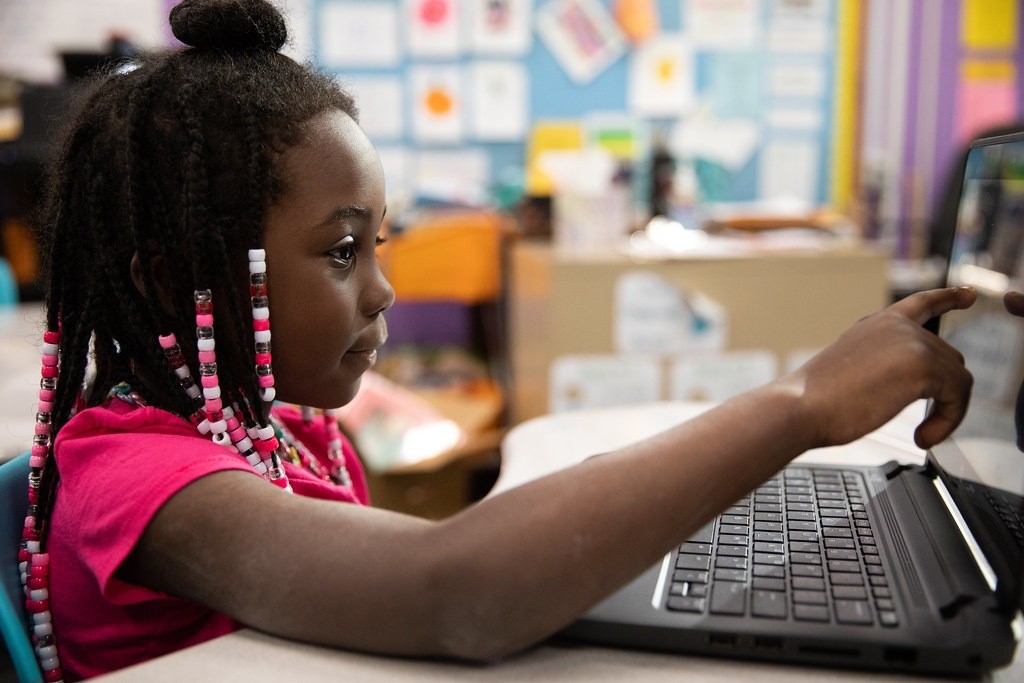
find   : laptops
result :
[550,122,1024,680]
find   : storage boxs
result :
[485,231,884,422]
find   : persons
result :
[18,0,978,683]
[466,196,551,362]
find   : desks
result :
[84,401,1024,683]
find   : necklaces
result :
[106,382,301,468]
